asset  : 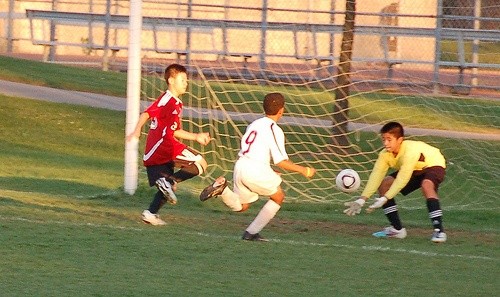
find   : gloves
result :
[343,198,364,216]
[366,197,387,213]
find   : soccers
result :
[335,168,360,193]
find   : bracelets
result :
[306,167,311,176]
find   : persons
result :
[125,64,211,226]
[342,121,447,243]
[200,92,317,242]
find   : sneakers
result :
[141,210,167,225]
[200,177,227,201]
[155,178,177,205]
[431,232,447,242]
[242,231,268,241]
[372,226,407,239]
